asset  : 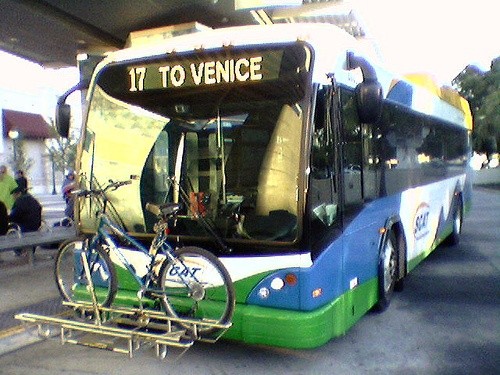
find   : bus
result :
[56,21,473,349]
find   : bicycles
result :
[53,180,235,338]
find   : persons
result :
[14,170,28,191]
[52,170,75,228]
[0,165,19,212]
[8,186,42,232]
